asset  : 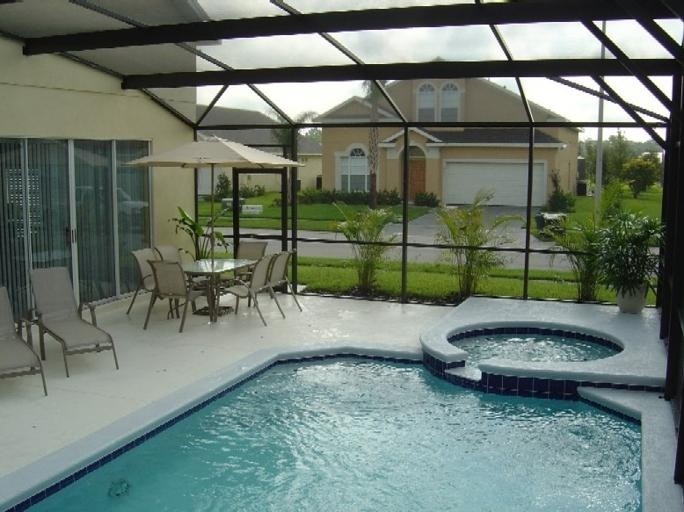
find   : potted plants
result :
[563,199,666,315]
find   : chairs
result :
[123,237,303,334]
[0,264,121,397]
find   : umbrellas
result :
[121,131,306,261]
